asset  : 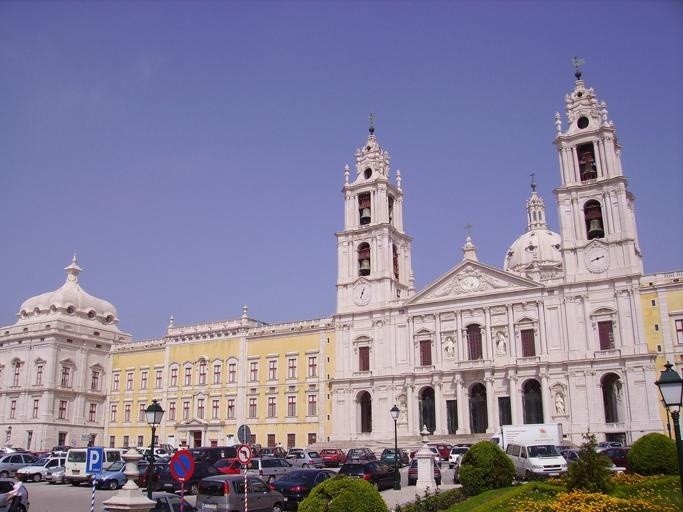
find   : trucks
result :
[487,422,565,452]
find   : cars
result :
[560,439,632,474]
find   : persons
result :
[4,474,29,512]
[554,393,565,415]
[495,331,506,353]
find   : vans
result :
[502,440,568,481]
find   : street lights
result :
[653,359,683,494]
[143,397,166,500]
[389,404,402,490]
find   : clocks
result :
[583,244,614,277]
[348,281,370,305]
